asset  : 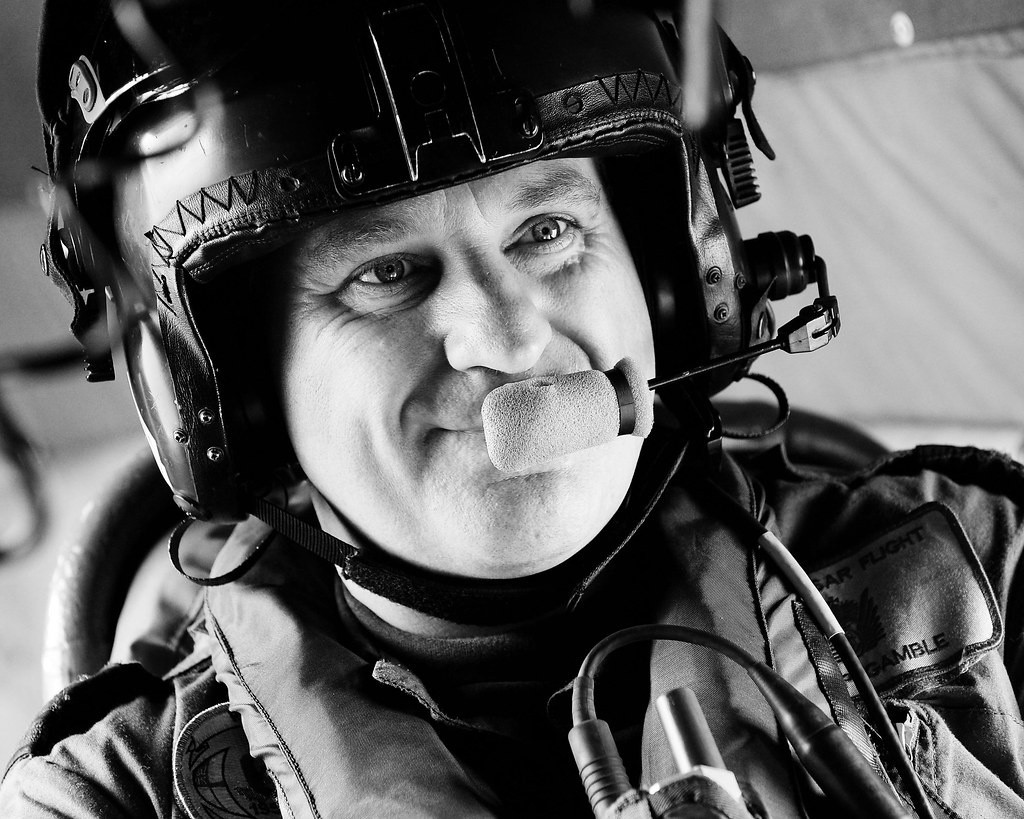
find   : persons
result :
[0,0,1024,819]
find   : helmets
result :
[39,0,842,524]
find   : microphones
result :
[480,230,840,473]
